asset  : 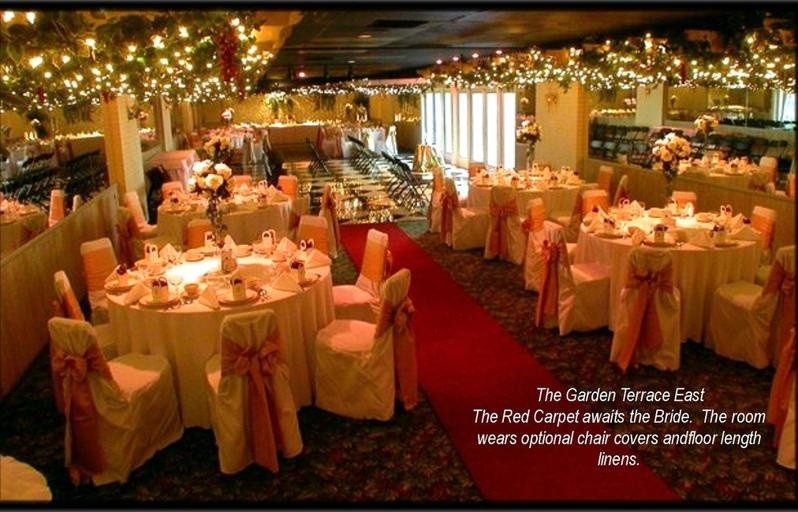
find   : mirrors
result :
[587,76,798,196]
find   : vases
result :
[523,142,536,178]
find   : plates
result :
[139,292,180,307]
[183,254,204,261]
[103,275,144,291]
[216,289,258,305]
[223,291,253,301]
[145,294,176,303]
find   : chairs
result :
[588,95,795,192]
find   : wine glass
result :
[168,270,183,301]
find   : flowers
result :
[217,104,236,124]
[139,110,150,124]
[513,119,544,150]
